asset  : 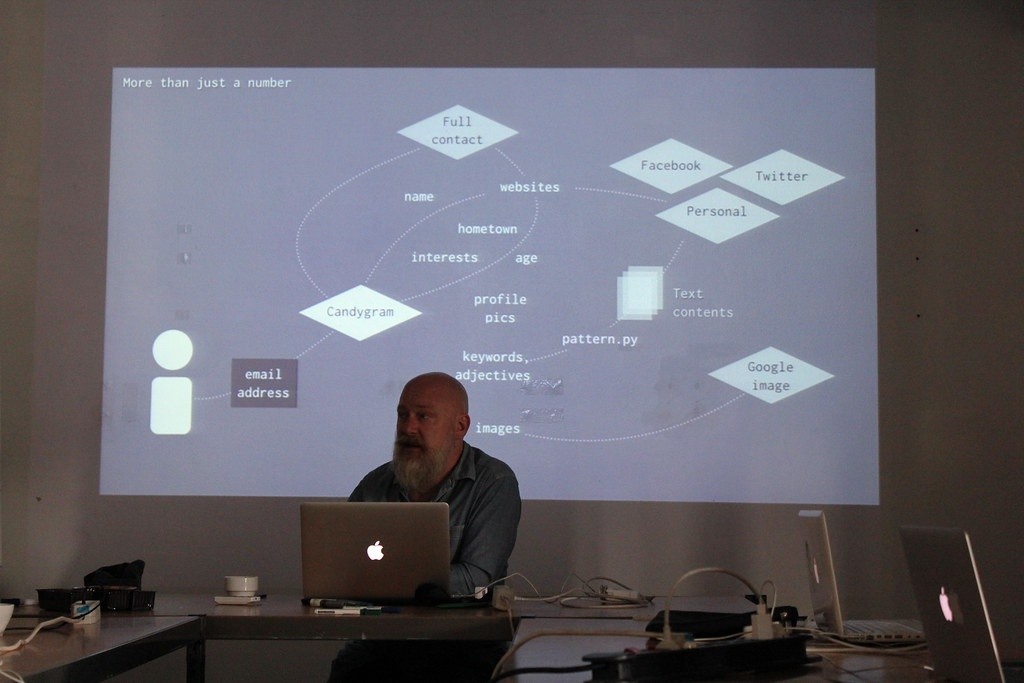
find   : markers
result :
[341,604,403,613]
[315,609,381,617]
[300,597,370,607]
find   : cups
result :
[225,574,259,599]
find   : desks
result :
[22,594,759,642]
[498,618,1023,683]
[1,618,205,683]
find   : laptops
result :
[299,501,452,602]
[898,525,1024,683]
[798,509,928,644]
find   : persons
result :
[326,371,523,683]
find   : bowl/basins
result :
[1,604,15,636]
